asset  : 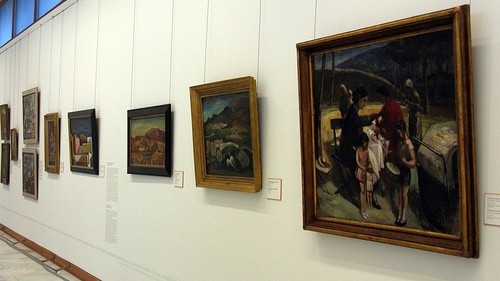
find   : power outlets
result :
[173,170,184,188]
[59,161,65,174]
[98,163,106,179]
[265,178,283,201]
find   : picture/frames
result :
[0,142,11,185]
[43,111,61,175]
[126,103,173,178]
[188,74,263,194]
[0,104,10,141]
[293,3,480,259]
[21,86,39,145]
[10,127,18,162]
[67,107,100,176]
[21,147,38,201]
